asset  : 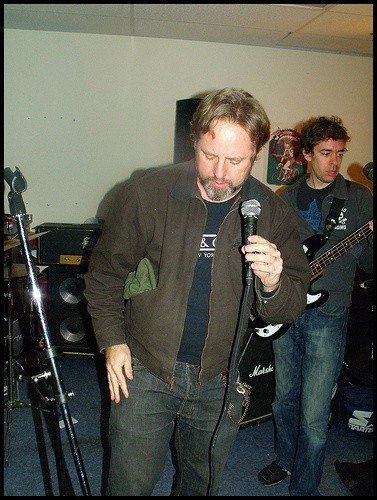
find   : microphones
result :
[241,199,264,284]
[364,162,373,180]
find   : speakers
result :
[36,223,103,266]
[235,329,275,430]
[45,267,101,357]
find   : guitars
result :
[254,219,372,340]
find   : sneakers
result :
[257,462,290,487]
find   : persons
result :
[85,86,311,496]
[256,116,374,496]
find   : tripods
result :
[3,231,62,468]
